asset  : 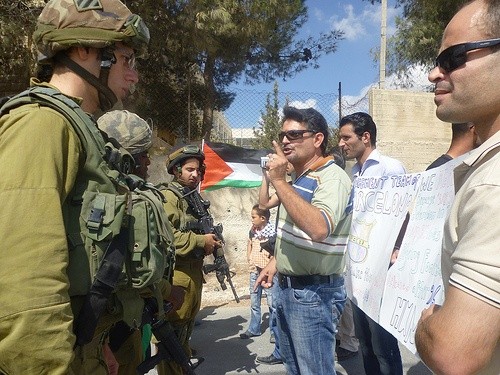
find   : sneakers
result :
[270,334,276,343]
[240,329,255,338]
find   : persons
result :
[157,145,222,375]
[240,204,277,342]
[337,112,403,375]
[414,0,500,375]
[327,150,359,361]
[259,108,354,375]
[0,0,175,375]
[426,121,477,171]
[97,110,153,181]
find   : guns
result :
[137,298,205,375]
[183,187,240,303]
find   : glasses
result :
[434,39,500,73]
[279,130,317,143]
[123,14,150,45]
[114,47,135,71]
[182,145,205,160]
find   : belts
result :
[278,273,339,288]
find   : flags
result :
[199,141,275,193]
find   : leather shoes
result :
[256,354,285,365]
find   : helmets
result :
[166,144,205,174]
[97,110,153,158]
[33,0,150,57]
